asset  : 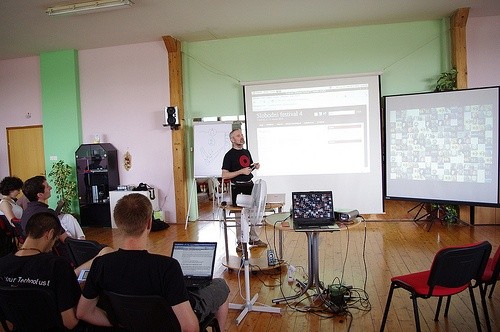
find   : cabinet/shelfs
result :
[75,143,121,228]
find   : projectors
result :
[334,209,359,222]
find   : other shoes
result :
[249,239,267,246]
[236,243,242,252]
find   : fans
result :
[231,178,283,324]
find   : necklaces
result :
[18,247,41,253]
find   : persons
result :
[222,129,267,251]
[0,172,229,332]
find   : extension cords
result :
[288,267,295,282]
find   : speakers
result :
[165,106,179,124]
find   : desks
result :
[265,210,361,313]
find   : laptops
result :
[292,191,341,232]
[171,242,217,289]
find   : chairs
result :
[444,245,500,324]
[0,215,18,256]
[64,236,102,267]
[102,289,221,332]
[0,286,86,332]
[380,239,492,332]
